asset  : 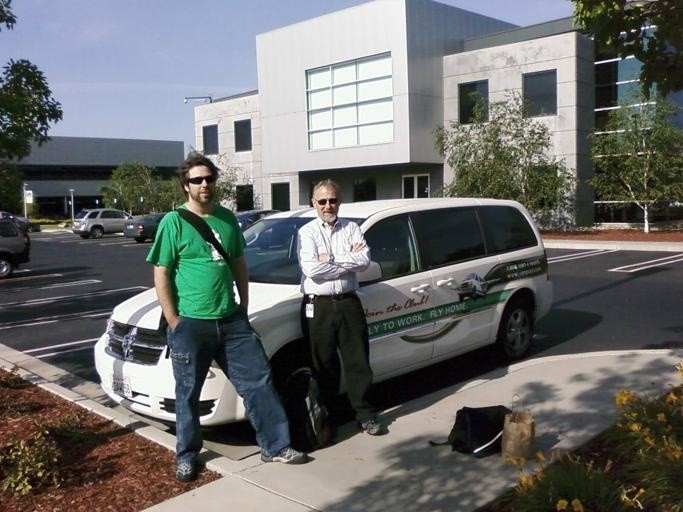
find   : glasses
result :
[186,174,216,184]
[314,198,338,205]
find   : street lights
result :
[68,188,75,223]
[22,182,29,219]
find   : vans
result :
[92,196,554,432]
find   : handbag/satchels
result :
[501,393,535,461]
[449,405,513,458]
[158,282,177,337]
[291,377,331,451]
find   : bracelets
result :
[329,253,335,263]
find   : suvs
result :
[0,211,30,279]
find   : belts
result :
[302,291,355,302]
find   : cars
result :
[71,207,131,239]
[232,207,291,248]
[122,211,165,243]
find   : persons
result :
[143,158,307,481]
[295,177,390,445]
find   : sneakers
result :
[361,418,383,434]
[176,461,194,482]
[261,446,308,465]
